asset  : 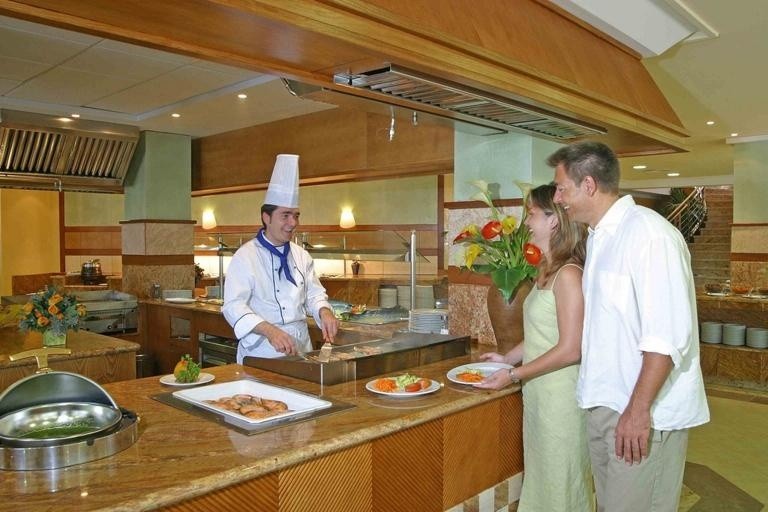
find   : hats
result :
[264,154,300,209]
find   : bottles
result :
[351,260,360,275]
[151,284,163,305]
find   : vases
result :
[42,330,67,346]
[486,277,535,356]
[351,262,359,275]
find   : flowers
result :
[452,180,542,302]
[16,285,87,336]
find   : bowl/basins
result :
[0,402,126,444]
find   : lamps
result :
[340,206,356,229]
[201,209,217,230]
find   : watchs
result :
[509,368,521,385]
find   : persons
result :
[472,181,594,512]
[222,154,340,365]
[546,142,711,512]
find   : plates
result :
[174,380,334,424]
[366,376,443,398]
[0,410,152,472]
[377,284,437,307]
[409,309,449,332]
[159,373,215,385]
[445,362,520,389]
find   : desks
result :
[195,275,439,306]
[0,344,524,512]
[142,296,410,380]
[0,326,142,396]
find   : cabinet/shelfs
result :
[696,292,768,390]
